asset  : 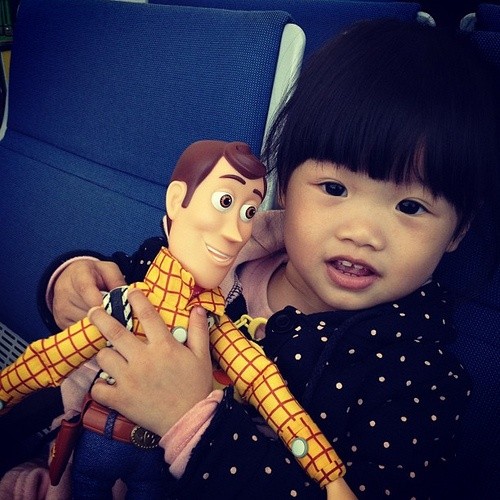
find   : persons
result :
[0,138,356,500]
[37,16,500,499]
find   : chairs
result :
[0,2,499,472]
